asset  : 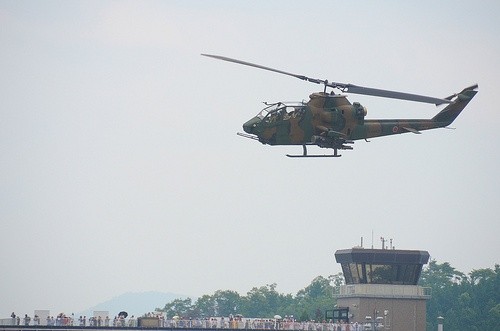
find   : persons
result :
[8,307,385,331]
[269,111,278,124]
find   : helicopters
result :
[199,53,478,158]
